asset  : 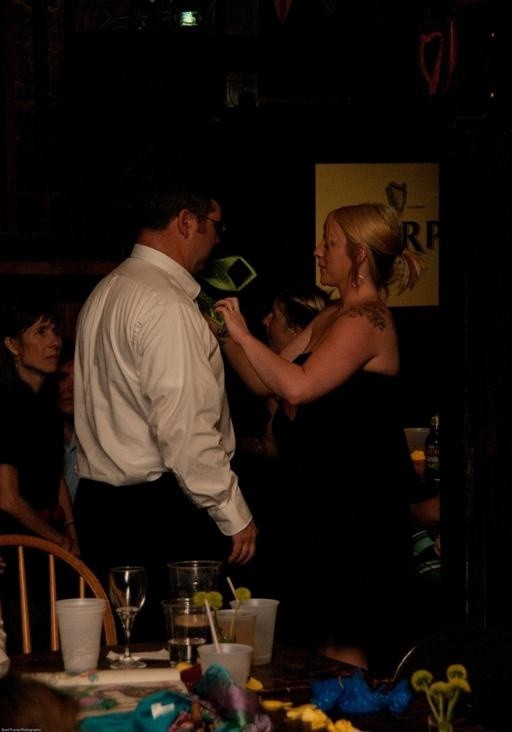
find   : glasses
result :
[196,214,227,234]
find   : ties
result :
[196,289,228,338]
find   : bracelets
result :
[64,520,75,531]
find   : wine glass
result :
[108,565,148,670]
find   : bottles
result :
[424,416,445,481]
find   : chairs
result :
[0,534,116,652]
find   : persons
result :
[201,199,418,646]
[62,175,270,642]
[44,355,88,503]
[258,289,326,456]
[404,472,446,590]
[0,304,83,655]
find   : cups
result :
[196,642,254,693]
[210,609,258,651]
[167,560,223,627]
[160,598,208,667]
[54,598,107,673]
[229,598,280,667]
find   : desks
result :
[0,642,512,731]
